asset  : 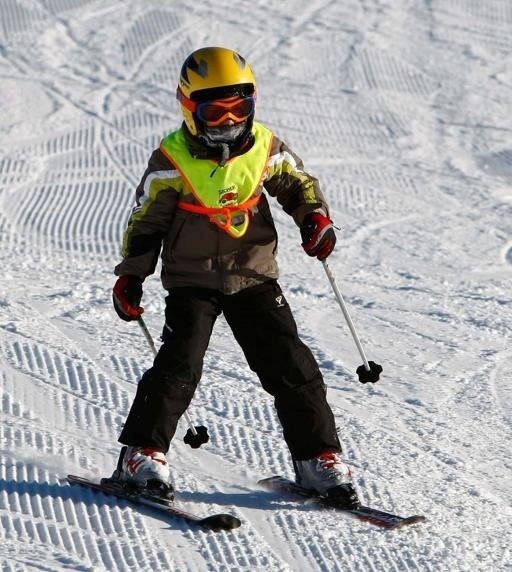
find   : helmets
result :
[175,48,257,159]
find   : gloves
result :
[113,275,144,322]
[297,213,336,261]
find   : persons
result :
[111,45,364,510]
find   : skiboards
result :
[66,475,430,530]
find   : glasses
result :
[176,85,256,130]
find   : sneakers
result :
[294,451,355,495]
[121,444,170,485]
